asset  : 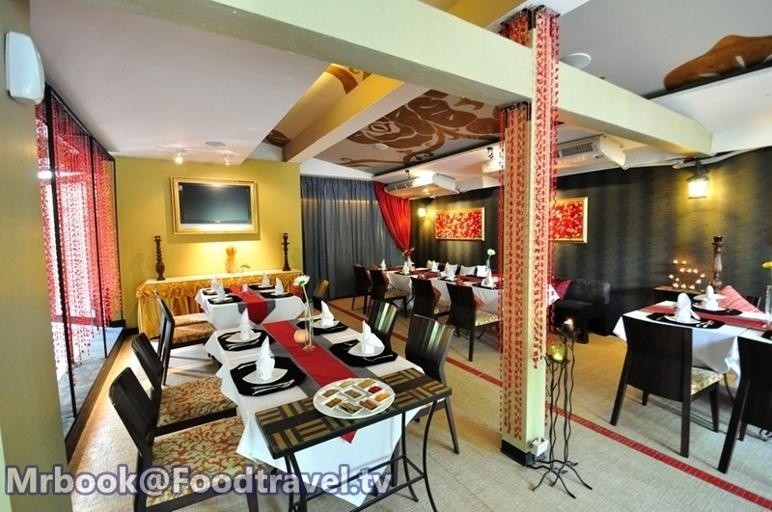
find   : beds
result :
[203,314,450,512]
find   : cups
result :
[675,302,692,322]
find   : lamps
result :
[686,158,709,199]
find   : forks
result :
[363,354,393,362]
[702,321,715,328]
[252,379,295,396]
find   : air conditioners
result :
[556,134,625,171]
[384,172,461,202]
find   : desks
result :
[136,269,306,340]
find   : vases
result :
[303,301,317,351]
[766,285,772,321]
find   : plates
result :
[313,377,395,419]
[203,282,386,384]
[693,303,726,312]
[664,315,701,324]
[378,265,497,288]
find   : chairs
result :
[130,332,237,435]
[405,314,460,456]
[716,336,772,473]
[351,260,611,360]
[108,368,271,512]
[609,316,719,458]
[155,296,214,384]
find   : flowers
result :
[762,261,772,313]
[292,275,311,346]
[487,248,495,272]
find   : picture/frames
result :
[435,208,484,242]
[552,197,588,244]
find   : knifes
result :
[696,322,708,327]
[250,382,287,390]
